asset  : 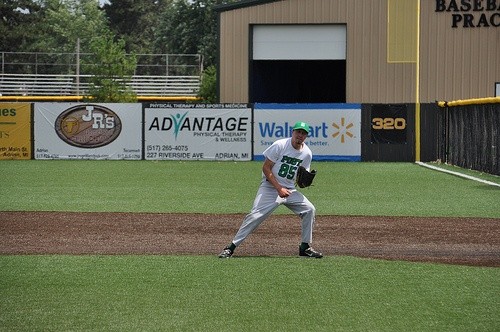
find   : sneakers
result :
[218,246,234,259]
[298,245,323,258]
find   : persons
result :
[217,120,324,259]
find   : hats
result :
[293,120,310,133]
